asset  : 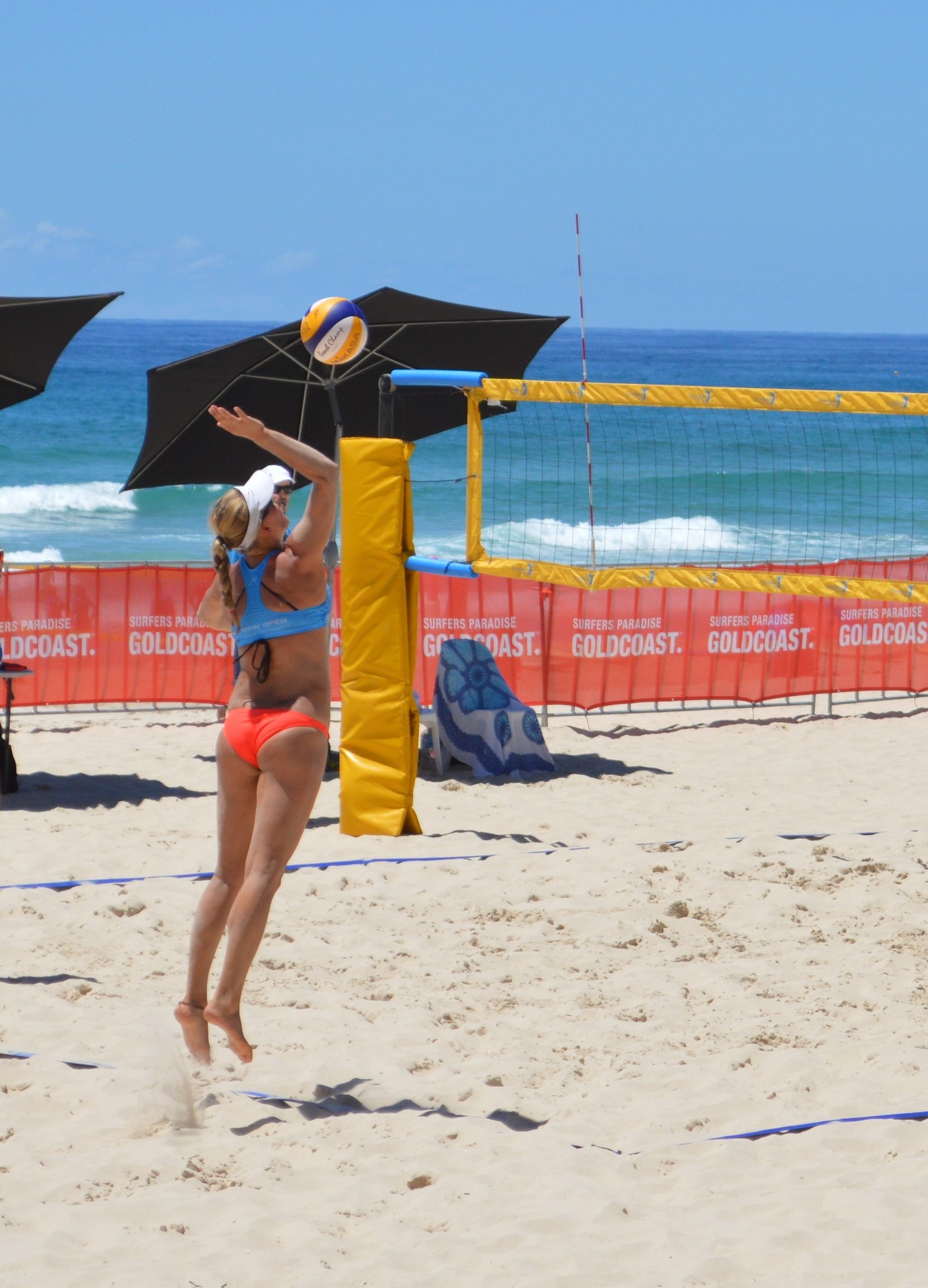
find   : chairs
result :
[433,639,556,778]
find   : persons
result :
[172,406,333,1067]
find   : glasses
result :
[273,484,293,494]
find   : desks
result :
[0,669,34,795]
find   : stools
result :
[417,708,443,776]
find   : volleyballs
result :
[300,297,369,364]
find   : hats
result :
[233,469,274,551]
[261,465,296,485]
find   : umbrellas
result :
[120,285,572,495]
[0,292,123,411]
